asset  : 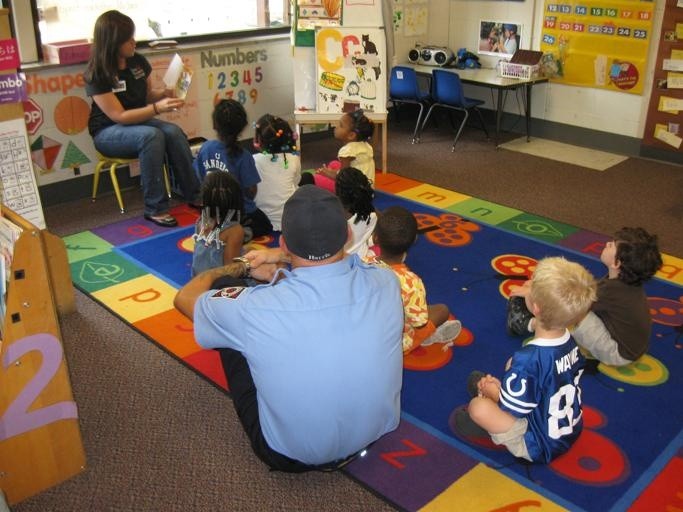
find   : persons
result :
[173,184,405,473]
[467,257,599,465]
[191,171,245,283]
[335,167,378,258]
[480,22,499,52]
[253,113,315,232]
[81,10,205,227]
[193,99,273,244]
[313,108,376,194]
[498,24,517,54]
[507,226,663,367]
[359,206,462,357]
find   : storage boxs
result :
[48,39,93,66]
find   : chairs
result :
[416,70,490,151]
[389,66,455,145]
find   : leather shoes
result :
[188,201,204,211]
[143,213,177,227]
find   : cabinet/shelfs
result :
[1,204,85,506]
[0,8,76,316]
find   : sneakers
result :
[454,410,490,438]
[466,371,485,397]
[506,296,535,335]
[420,319,462,346]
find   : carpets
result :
[62,172,683,512]
[497,135,629,171]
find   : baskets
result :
[500,61,539,80]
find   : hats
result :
[281,184,347,261]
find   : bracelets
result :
[232,256,251,279]
[153,102,160,115]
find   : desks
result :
[391,62,549,149]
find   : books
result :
[0,217,23,321]
[162,53,194,103]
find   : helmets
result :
[504,24,517,33]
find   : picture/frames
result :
[478,20,522,58]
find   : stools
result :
[91,152,171,214]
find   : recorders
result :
[407,45,454,67]
